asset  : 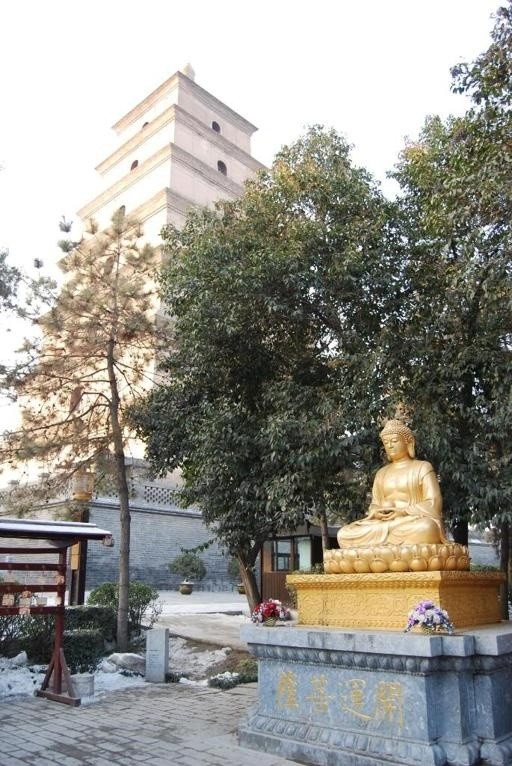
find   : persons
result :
[337,418,443,549]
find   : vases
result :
[262,616,277,627]
[420,622,436,635]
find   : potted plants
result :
[61,627,104,698]
[226,558,257,595]
[166,553,209,595]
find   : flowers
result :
[250,597,290,627]
[401,599,454,636]
